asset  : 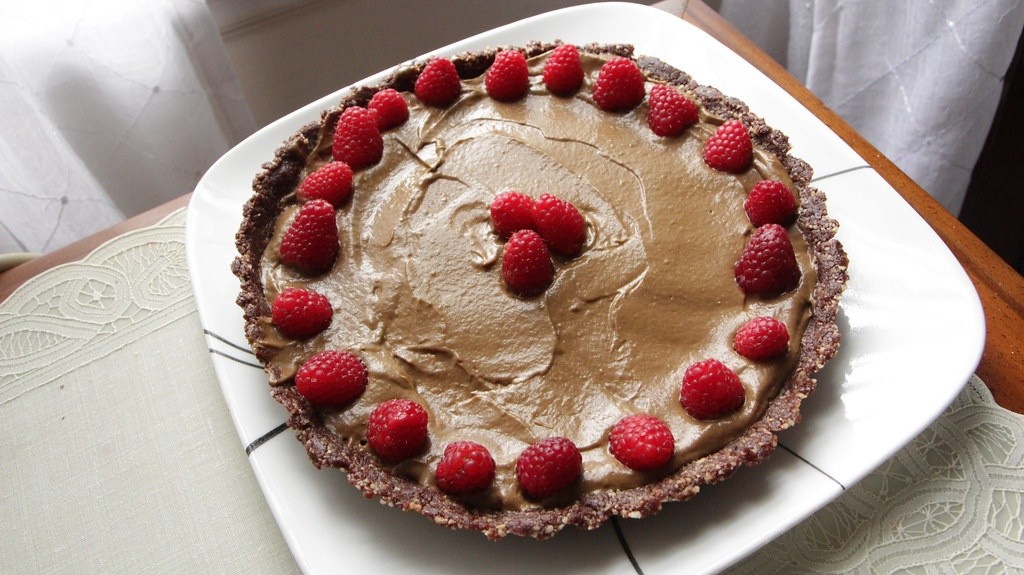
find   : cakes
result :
[233,43,853,541]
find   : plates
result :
[184,0,989,575]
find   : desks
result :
[1,0,1024,574]
